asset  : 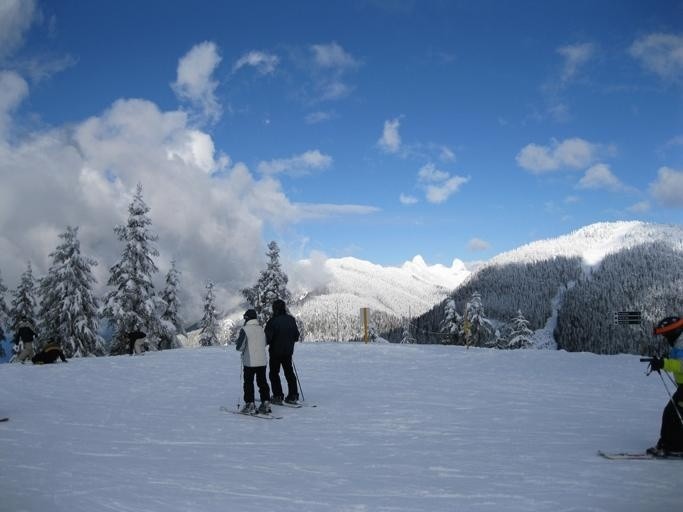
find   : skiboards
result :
[598,449,683,460]
[219,405,283,419]
[254,395,318,408]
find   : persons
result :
[263,299,299,405]
[636,316,682,456]
[232,307,273,416]
[10,314,38,362]
[122,330,147,356]
[27,341,69,364]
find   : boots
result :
[240,402,256,414]
[258,400,269,412]
[271,395,284,404]
[285,394,298,404]
[647,439,683,455]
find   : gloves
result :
[650,359,662,370]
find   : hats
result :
[273,300,285,311]
[244,309,257,319]
[656,316,683,334]
